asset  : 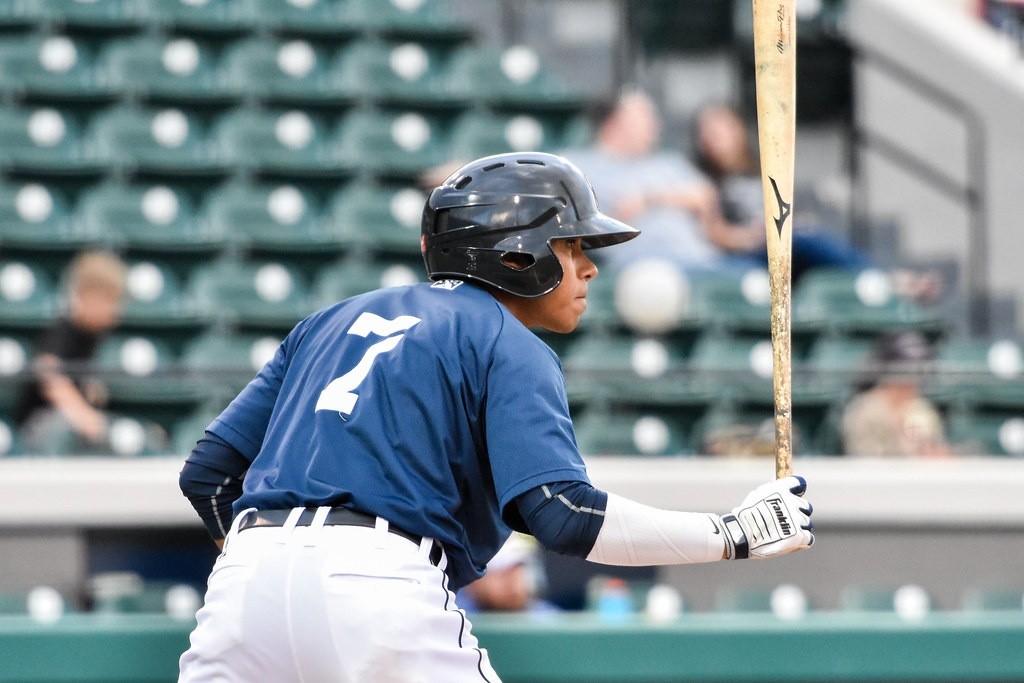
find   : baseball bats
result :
[753,0,796,482]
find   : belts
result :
[234,506,442,567]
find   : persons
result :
[445,531,663,612]
[837,328,985,455]
[7,251,167,458]
[178,150,816,683]
[556,86,960,307]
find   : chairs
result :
[0,0,1024,457]
[0,583,1024,683]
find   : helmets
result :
[421,152,639,300]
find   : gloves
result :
[719,474,815,561]
